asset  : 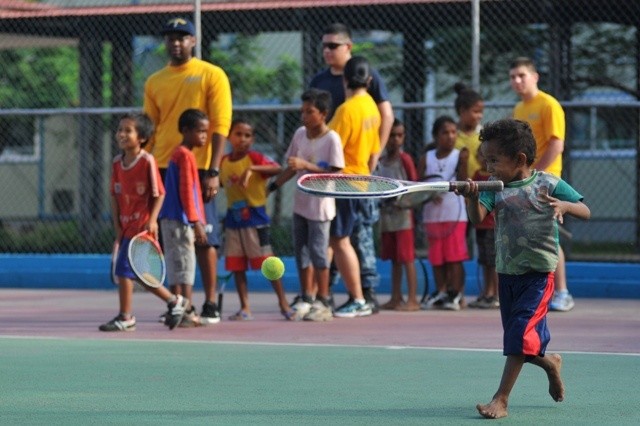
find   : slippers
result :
[227,310,254,321]
[280,307,298,321]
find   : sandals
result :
[378,295,406,312]
[395,299,422,312]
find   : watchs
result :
[208,168,219,177]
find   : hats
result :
[163,18,195,35]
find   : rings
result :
[213,189,217,194]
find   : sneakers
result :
[178,306,202,326]
[291,293,314,322]
[443,291,463,311]
[158,313,167,323]
[478,296,500,308]
[303,294,334,321]
[419,291,451,308]
[367,298,379,314]
[468,296,484,307]
[333,296,373,317]
[548,288,574,311]
[99,314,137,331]
[168,295,190,330]
[199,300,222,323]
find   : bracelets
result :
[268,182,278,191]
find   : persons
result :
[308,23,394,313]
[468,144,502,309]
[452,82,486,178]
[417,115,468,310]
[509,57,576,311]
[220,118,299,321]
[375,118,420,311]
[143,14,232,325]
[455,119,590,420]
[325,54,381,318]
[97,111,189,331]
[263,86,346,323]
[158,108,209,329]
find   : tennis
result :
[261,256,285,280]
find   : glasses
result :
[323,43,351,50]
